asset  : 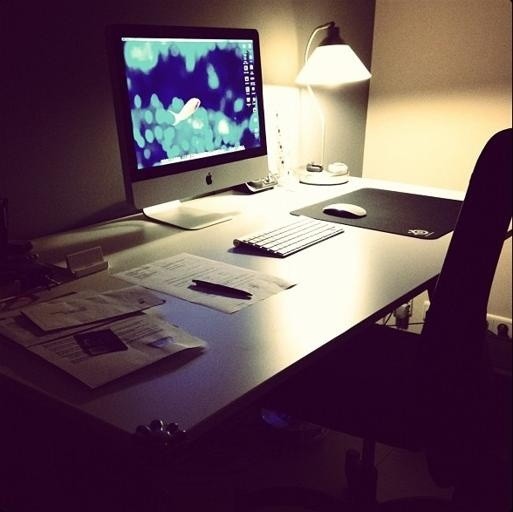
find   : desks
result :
[1,171,513,512]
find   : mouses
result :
[322,203,367,219]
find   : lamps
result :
[294,22,373,185]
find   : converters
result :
[395,304,410,319]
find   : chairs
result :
[249,129,513,499]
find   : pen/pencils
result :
[192,280,253,297]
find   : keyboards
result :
[232,214,344,257]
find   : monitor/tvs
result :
[107,24,270,231]
